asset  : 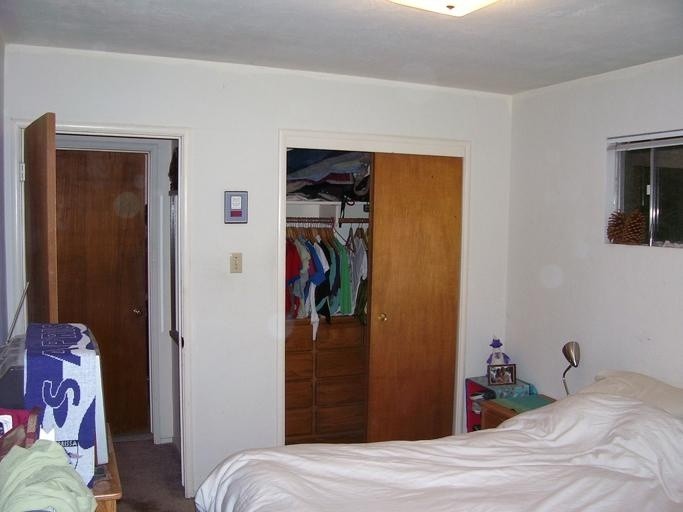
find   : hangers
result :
[285,216,370,250]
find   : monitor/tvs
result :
[0,322,109,467]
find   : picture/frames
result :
[488,364,516,386]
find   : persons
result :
[501,367,512,384]
[486,338,510,377]
[491,368,503,384]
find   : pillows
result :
[578,371,683,418]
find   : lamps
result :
[561,342,579,395]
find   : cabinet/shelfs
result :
[466,379,533,433]
[479,395,558,429]
[284,313,366,445]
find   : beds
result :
[194,371,683,512]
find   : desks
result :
[88,422,122,512]
